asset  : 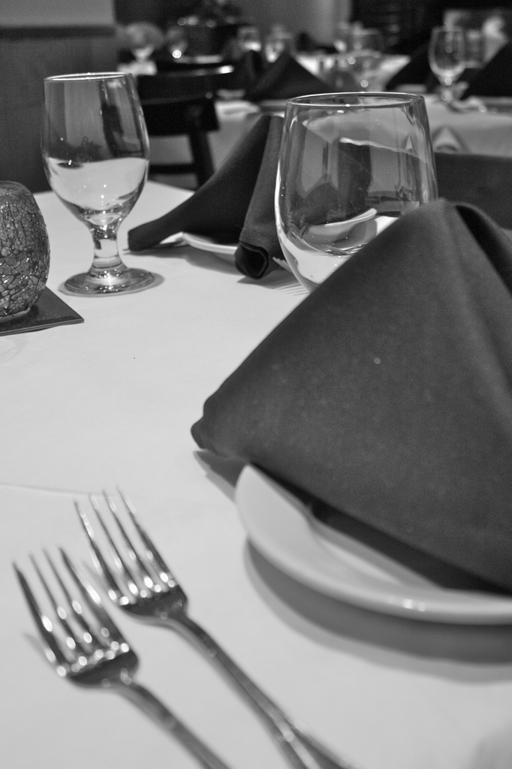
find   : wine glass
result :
[333,21,362,56]
[429,26,465,110]
[345,29,385,91]
[126,22,155,75]
[237,27,262,53]
[275,90,441,291]
[266,32,297,62]
[40,71,165,296]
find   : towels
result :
[126,113,375,281]
[189,199,511,593]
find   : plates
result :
[183,207,382,271]
[235,462,511,626]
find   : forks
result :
[72,486,350,769]
[14,546,231,768]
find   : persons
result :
[208,3,248,55]
